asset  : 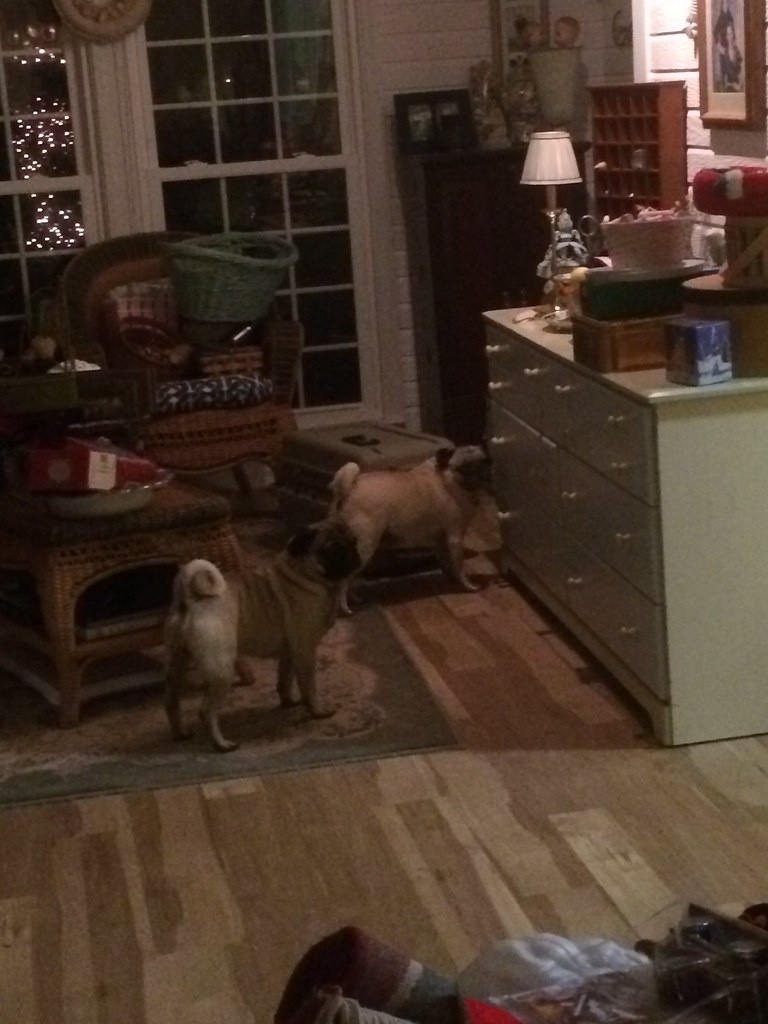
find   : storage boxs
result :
[570,258,732,388]
[276,419,455,579]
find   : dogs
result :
[310,437,492,618]
[161,516,365,751]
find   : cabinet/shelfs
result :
[395,140,592,464]
[481,306,768,746]
[584,80,689,226]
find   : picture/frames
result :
[489,0,552,86]
[698,0,767,134]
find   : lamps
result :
[520,131,583,317]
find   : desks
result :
[0,450,256,732]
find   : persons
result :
[710,0,742,92]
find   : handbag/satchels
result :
[454,931,678,1024]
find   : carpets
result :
[0,485,466,811]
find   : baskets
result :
[602,214,695,270]
[153,232,297,323]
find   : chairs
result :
[59,229,300,517]
